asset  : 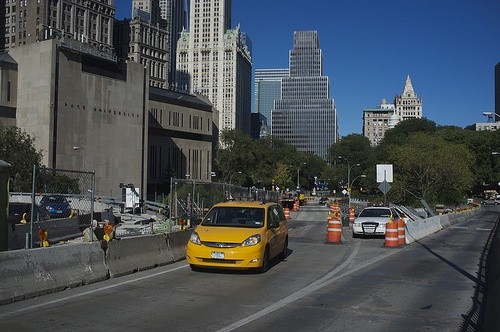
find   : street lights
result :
[337,156,361,207]
[298,163,307,191]
[229,171,242,198]
[350,175,367,197]
[72,146,86,171]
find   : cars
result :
[10,201,45,222]
[38,195,72,218]
[481,200,498,206]
[494,197,500,204]
[351,206,398,238]
[318,198,330,206]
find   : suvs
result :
[186,200,289,272]
